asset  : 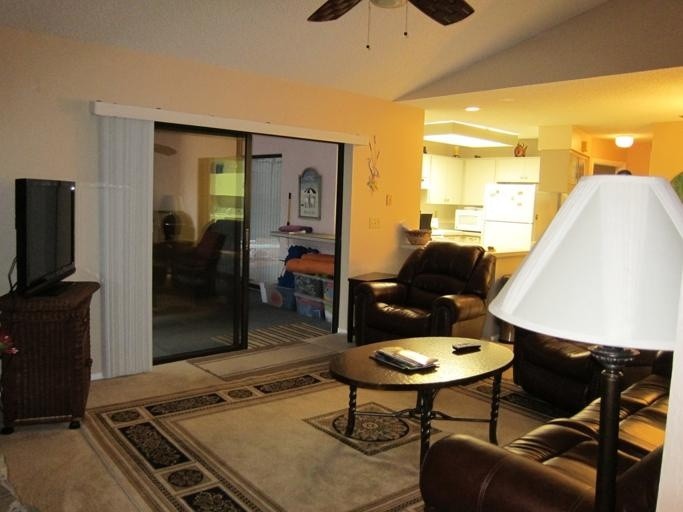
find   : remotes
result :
[452,341,481,350]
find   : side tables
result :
[347,272,398,343]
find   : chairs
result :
[172,224,226,297]
[354,241,496,345]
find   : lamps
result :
[488,173,683,512]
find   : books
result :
[368,347,440,370]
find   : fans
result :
[307,1,475,27]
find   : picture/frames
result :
[297,167,322,220]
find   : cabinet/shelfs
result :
[422,154,496,207]
[0,282,101,434]
[495,157,540,184]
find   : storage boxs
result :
[267,273,334,324]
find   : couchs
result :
[419,351,673,512]
[513,324,658,411]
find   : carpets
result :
[188,340,337,381]
[212,321,328,350]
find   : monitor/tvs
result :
[15,178,75,296]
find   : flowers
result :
[0,336,19,369]
